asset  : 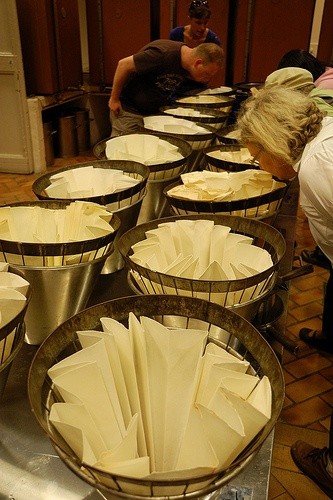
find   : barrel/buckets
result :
[42,108,90,166]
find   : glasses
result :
[189,0,210,9]
[249,145,264,166]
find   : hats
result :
[264,67,316,91]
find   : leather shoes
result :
[301,244,332,270]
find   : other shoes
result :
[299,327,331,357]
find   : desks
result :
[0,138,300,500]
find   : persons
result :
[108,39,224,138]
[238,49,333,500]
[170,0,221,96]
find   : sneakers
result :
[290,439,333,500]
